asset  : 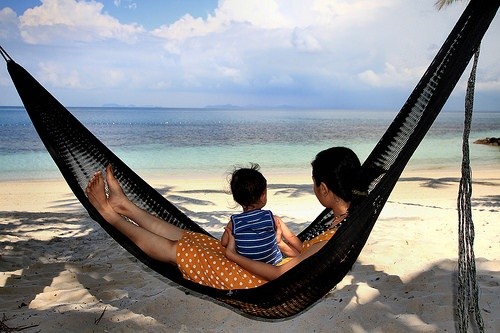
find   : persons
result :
[221,162,303,267]
[87,147,369,317]
